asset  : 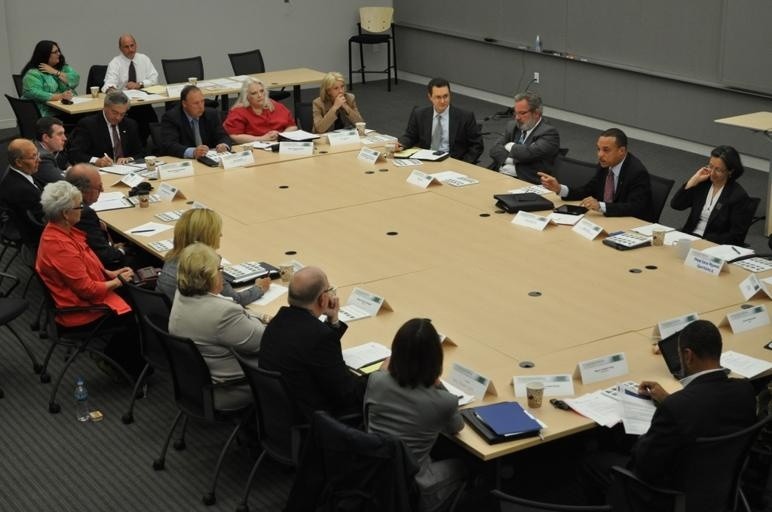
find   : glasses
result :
[70,204,84,212]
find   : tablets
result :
[553,203,587,216]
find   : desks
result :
[62,125,771,465]
[47,66,326,129]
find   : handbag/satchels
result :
[494,193,553,213]
[132,266,162,287]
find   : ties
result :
[192,119,202,146]
[129,61,136,82]
[111,125,125,160]
[604,169,614,203]
[431,116,442,150]
[519,132,525,143]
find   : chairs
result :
[21,243,144,415]
[228,346,363,512]
[141,314,254,506]
[227,48,291,102]
[162,55,220,113]
[115,272,192,452]
[488,489,611,512]
[0,63,108,401]
[311,407,475,512]
[606,415,771,510]
[345,5,400,93]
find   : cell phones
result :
[432,151,445,156]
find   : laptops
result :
[658,332,684,381]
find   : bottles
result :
[536,35,544,53]
[74,376,91,422]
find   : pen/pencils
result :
[399,146,407,147]
[732,247,740,254]
[131,229,154,233]
[125,197,135,207]
[265,269,270,278]
[104,153,109,158]
[646,386,651,392]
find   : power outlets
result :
[533,72,540,84]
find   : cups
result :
[383,143,396,161]
[525,381,545,410]
[673,238,692,258]
[355,122,366,137]
[652,226,667,247]
[144,156,157,172]
[188,77,198,86]
[137,186,149,210]
[243,143,254,154]
[90,86,100,98]
[279,263,296,283]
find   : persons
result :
[258,268,360,425]
[362,315,476,512]
[66,163,152,271]
[101,35,159,144]
[21,38,80,118]
[538,128,653,225]
[489,93,560,185]
[161,85,231,159]
[671,145,751,244]
[392,78,484,164]
[35,178,154,378]
[222,74,298,144]
[590,319,756,511]
[170,244,266,410]
[69,88,144,167]
[2,115,67,247]
[313,71,364,133]
[155,207,269,305]
